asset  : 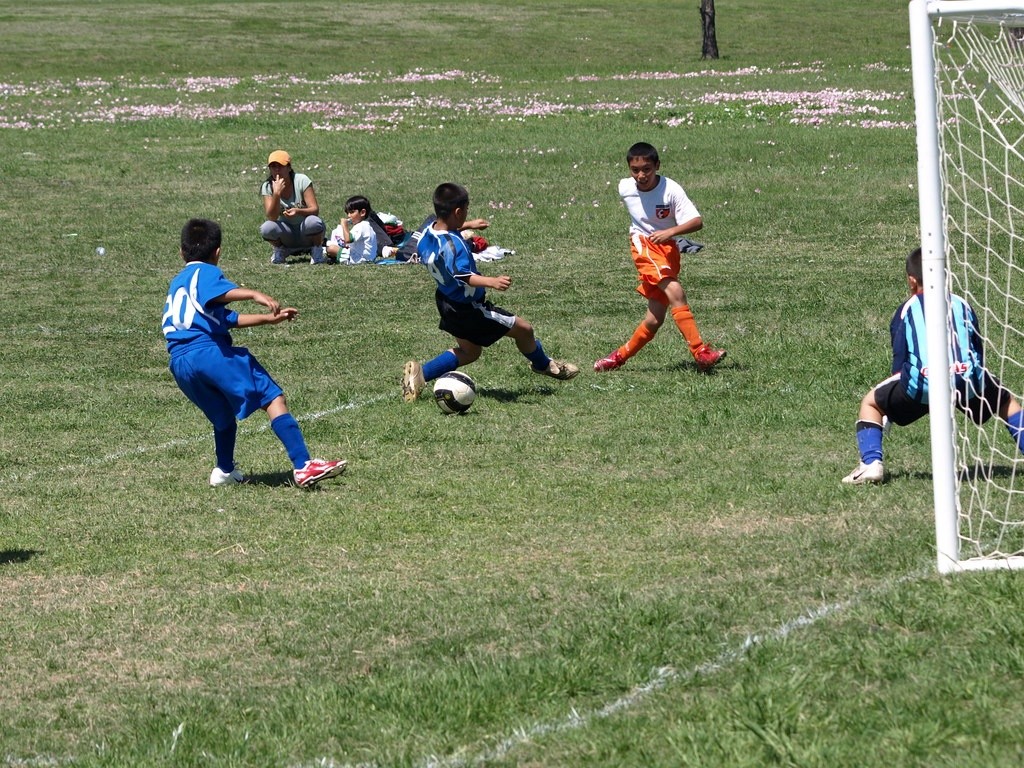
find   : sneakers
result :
[310,246,323,264]
[697,341,726,370]
[842,460,884,484]
[293,457,348,488]
[401,361,426,404]
[594,350,626,372]
[271,246,289,264]
[531,357,580,380]
[210,464,243,488]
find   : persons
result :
[161,218,349,487]
[593,142,728,371]
[260,150,325,263]
[840,247,1024,485]
[401,184,580,405]
[326,194,378,265]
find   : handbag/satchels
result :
[365,211,393,257]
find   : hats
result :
[268,150,291,167]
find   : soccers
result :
[432,370,476,414]
